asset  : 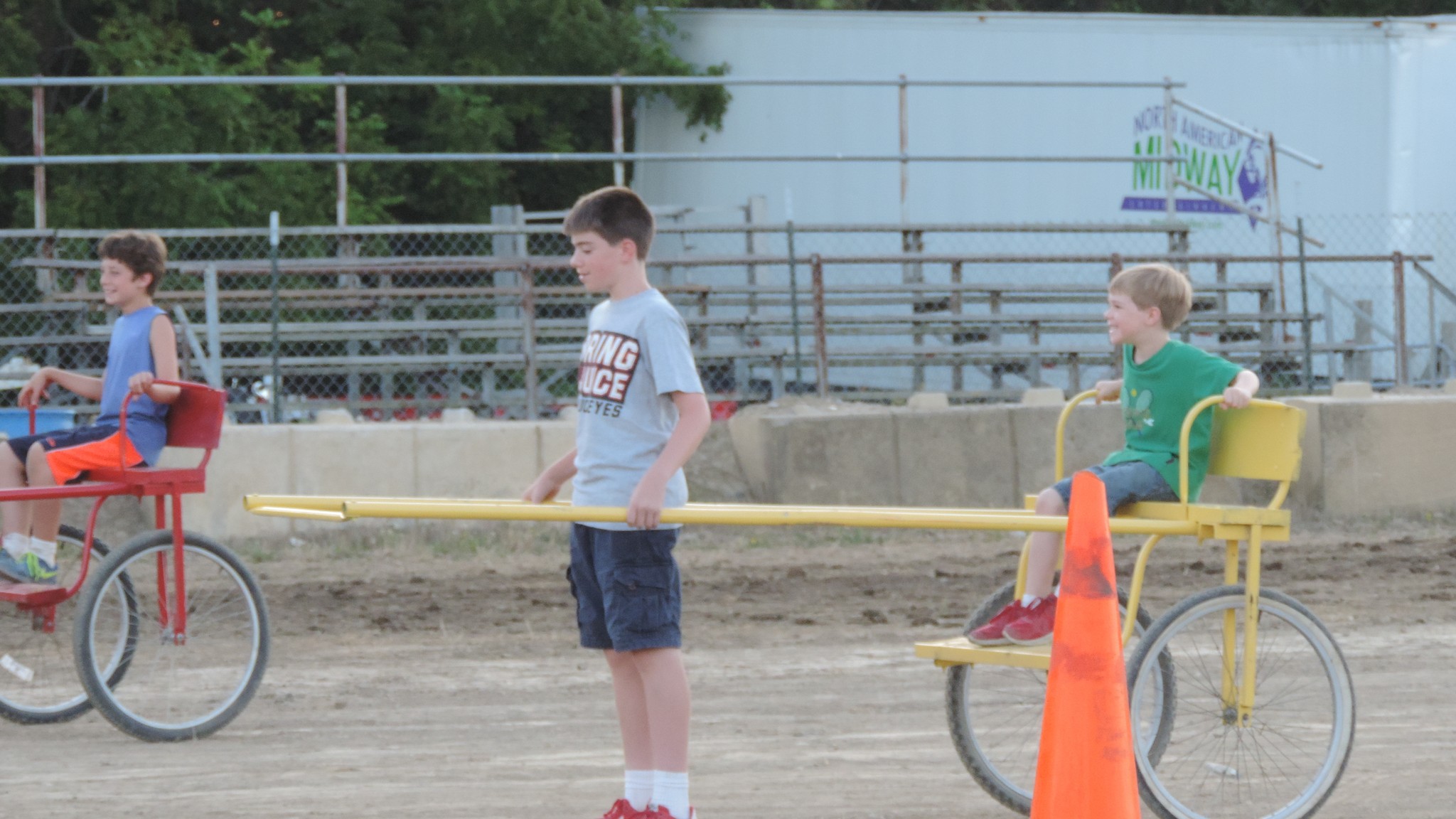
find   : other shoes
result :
[601,798,696,819]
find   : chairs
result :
[1023,390,1306,526]
[29,374,227,485]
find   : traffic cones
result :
[1028,470,1144,819]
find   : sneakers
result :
[1003,592,1058,646]
[0,549,57,585]
[968,599,1032,647]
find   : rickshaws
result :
[1,374,268,745]
[245,379,1355,818]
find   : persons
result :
[966,264,1260,645]
[0,231,181,586]
[521,187,712,819]
